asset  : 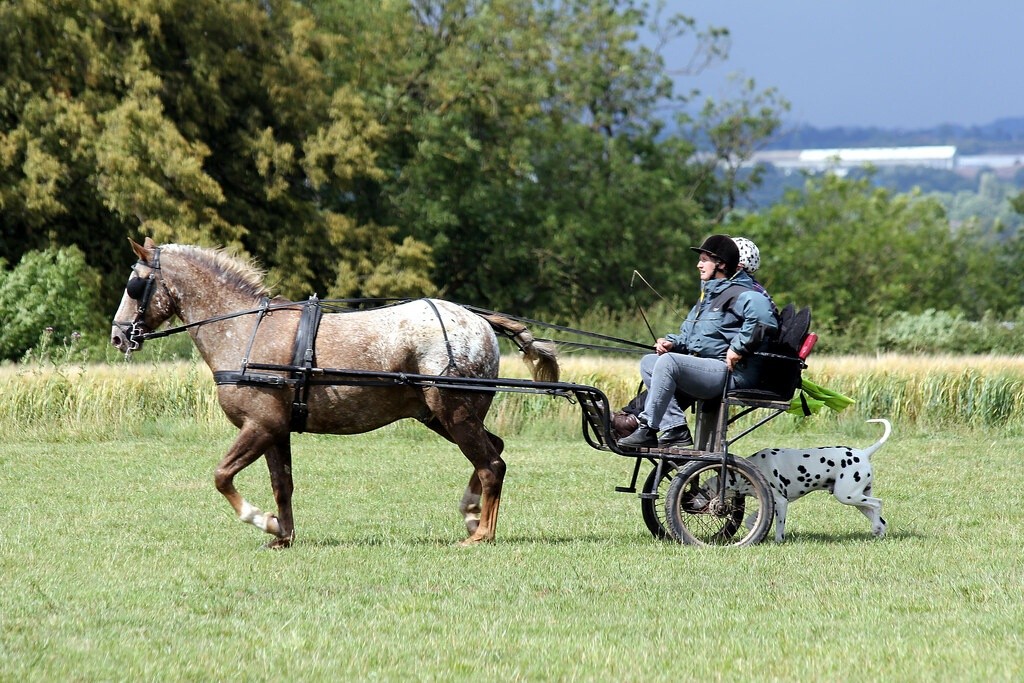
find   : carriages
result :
[108,236,854,549]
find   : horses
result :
[108,235,561,550]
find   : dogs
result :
[680,419,892,545]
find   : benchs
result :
[694,303,818,453]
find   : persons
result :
[604,237,779,438]
[594,236,780,450]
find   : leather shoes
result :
[616,425,658,448]
[611,411,639,438]
[657,428,693,447]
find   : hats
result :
[689,235,739,268]
[730,237,760,272]
